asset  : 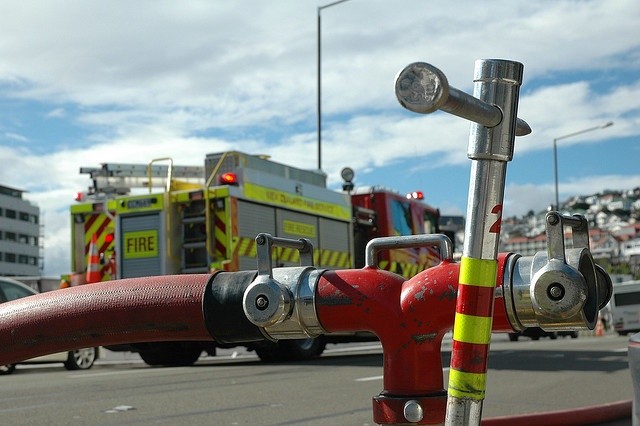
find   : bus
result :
[610,278,640,336]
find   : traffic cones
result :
[595,310,603,336]
[84,232,103,283]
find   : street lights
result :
[553,120,614,209]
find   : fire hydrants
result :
[243,211,613,426]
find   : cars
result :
[508,326,579,341]
[0,275,98,375]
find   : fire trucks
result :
[61,149,456,367]
